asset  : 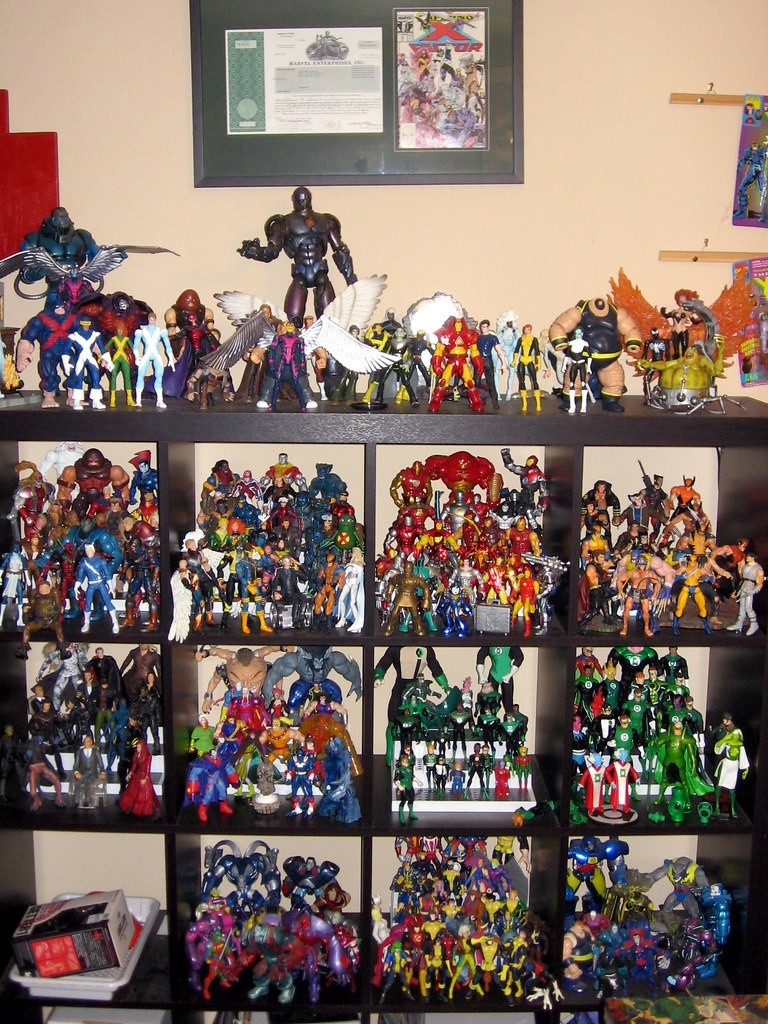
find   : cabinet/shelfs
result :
[0,391,767,1024]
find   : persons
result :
[0,448,763,1002]
[16,187,724,412]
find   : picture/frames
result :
[188,0,525,188]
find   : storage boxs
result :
[12,889,135,979]
